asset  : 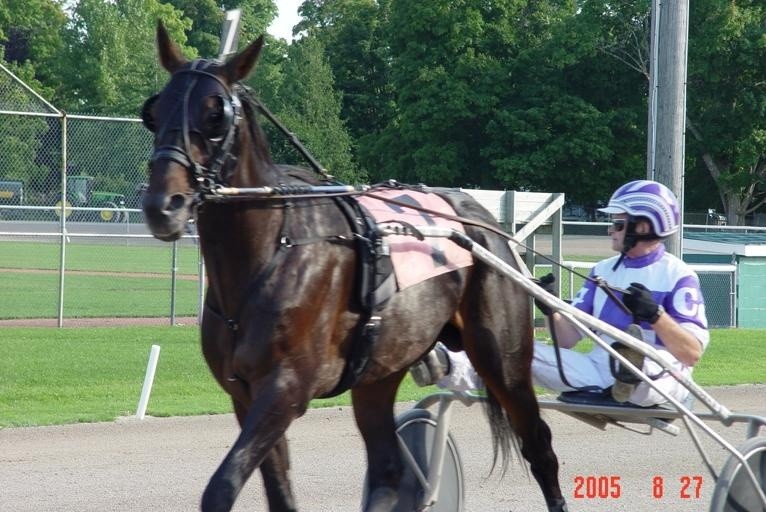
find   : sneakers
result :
[610,321,645,404]
[411,345,451,388]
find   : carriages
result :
[129,16,766,512]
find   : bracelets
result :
[648,304,664,324]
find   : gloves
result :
[623,283,664,324]
[532,272,560,315]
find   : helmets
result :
[596,180,682,238]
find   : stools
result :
[554,382,696,436]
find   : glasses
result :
[610,218,627,233]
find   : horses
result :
[137,18,566,512]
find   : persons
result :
[410,179,712,410]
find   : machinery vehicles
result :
[49,173,131,225]
